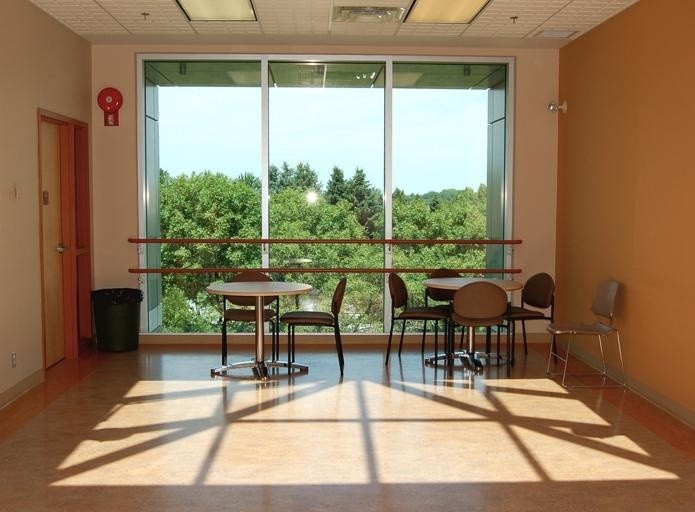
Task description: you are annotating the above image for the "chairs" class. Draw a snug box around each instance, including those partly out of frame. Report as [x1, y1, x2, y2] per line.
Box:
[546, 279, 626, 388]
[385, 268, 558, 376]
[222, 271, 347, 376]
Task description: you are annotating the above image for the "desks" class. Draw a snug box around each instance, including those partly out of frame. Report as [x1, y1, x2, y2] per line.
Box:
[206, 282, 313, 381]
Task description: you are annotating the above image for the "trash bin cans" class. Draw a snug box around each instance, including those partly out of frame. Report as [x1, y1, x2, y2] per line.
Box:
[91, 288, 144, 352]
